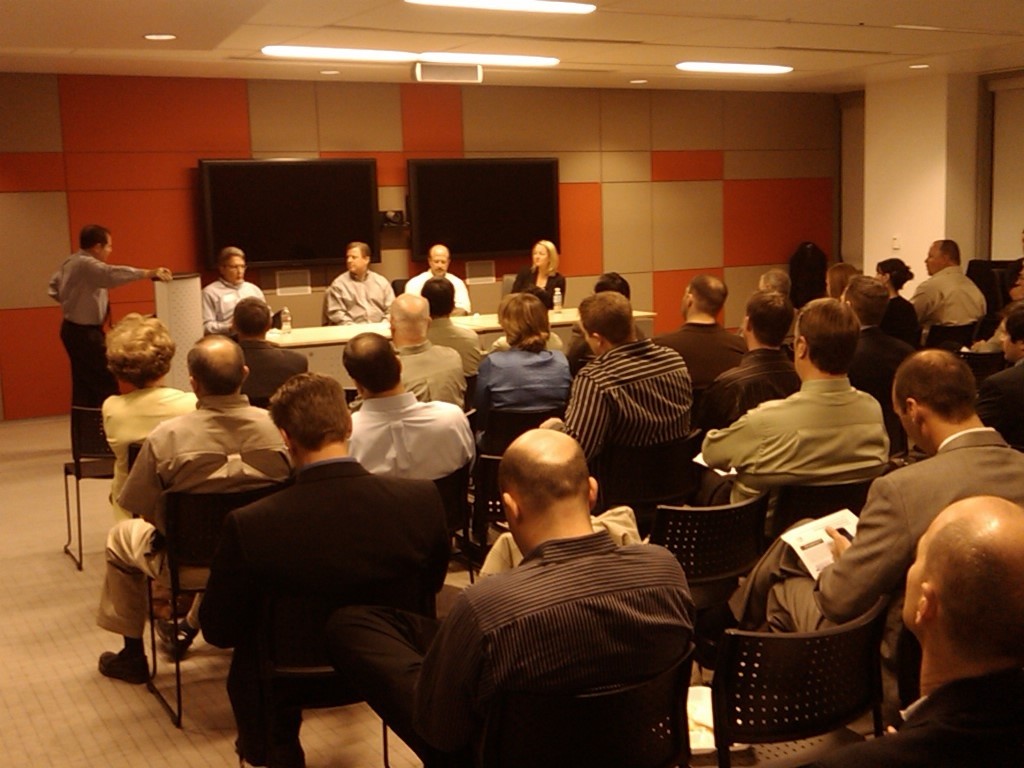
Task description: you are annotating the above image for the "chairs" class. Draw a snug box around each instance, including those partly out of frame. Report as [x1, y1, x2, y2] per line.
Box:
[64, 258, 1023, 768]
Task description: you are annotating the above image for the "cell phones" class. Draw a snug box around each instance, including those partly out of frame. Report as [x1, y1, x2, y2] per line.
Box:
[838, 528, 852, 542]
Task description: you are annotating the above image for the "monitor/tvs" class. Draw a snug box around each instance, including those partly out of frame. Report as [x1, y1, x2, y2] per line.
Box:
[199, 157, 381, 268]
[408, 157, 561, 262]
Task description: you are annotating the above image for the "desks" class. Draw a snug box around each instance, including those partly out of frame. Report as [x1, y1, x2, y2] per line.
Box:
[264, 307, 658, 390]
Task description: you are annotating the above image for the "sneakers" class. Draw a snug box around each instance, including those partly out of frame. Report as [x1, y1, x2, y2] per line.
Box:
[155, 618, 192, 656]
[98, 649, 150, 683]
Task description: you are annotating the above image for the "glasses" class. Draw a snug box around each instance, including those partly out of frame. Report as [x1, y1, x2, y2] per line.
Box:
[787, 339, 796, 350]
[225, 263, 247, 271]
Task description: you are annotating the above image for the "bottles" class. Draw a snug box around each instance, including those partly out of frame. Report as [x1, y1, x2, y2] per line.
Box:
[553, 287, 563, 314]
[281, 306, 292, 334]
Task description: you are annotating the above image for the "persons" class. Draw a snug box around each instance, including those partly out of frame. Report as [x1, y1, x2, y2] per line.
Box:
[811, 496, 1024, 768]
[348, 259, 1024, 540]
[199, 371, 450, 768]
[232, 297, 308, 408]
[511, 240, 566, 310]
[907, 240, 987, 353]
[693, 350, 1024, 729]
[404, 245, 472, 317]
[101, 311, 201, 524]
[202, 247, 274, 345]
[342, 332, 476, 525]
[48, 223, 174, 409]
[324, 242, 397, 328]
[336, 428, 694, 768]
[96, 334, 295, 683]
